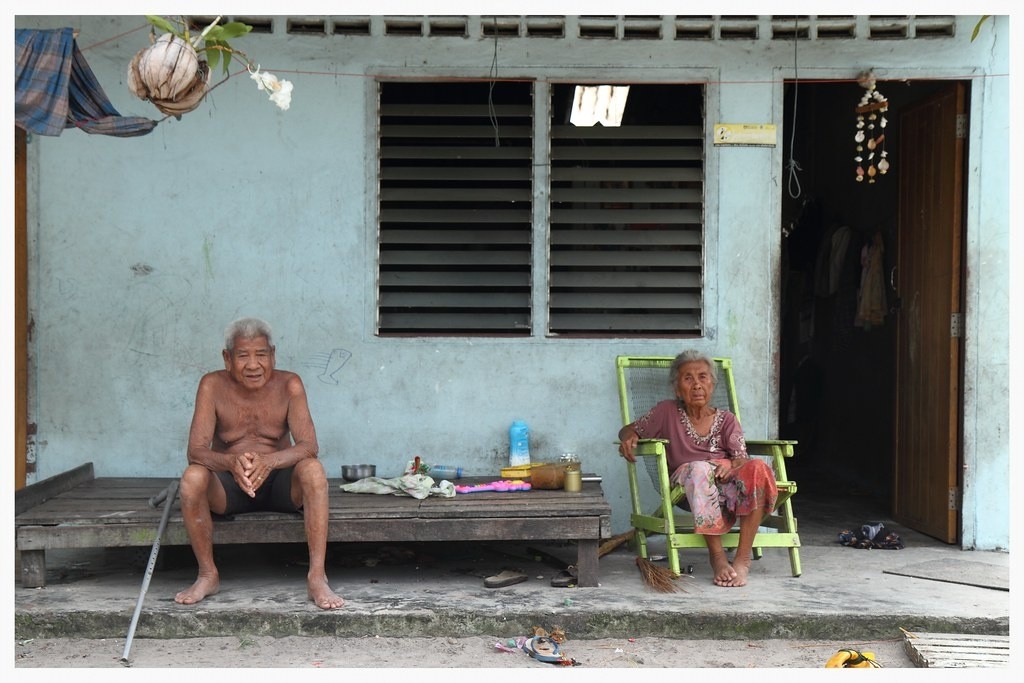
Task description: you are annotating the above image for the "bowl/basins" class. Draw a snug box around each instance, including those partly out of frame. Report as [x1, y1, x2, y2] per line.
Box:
[342, 464, 376, 482]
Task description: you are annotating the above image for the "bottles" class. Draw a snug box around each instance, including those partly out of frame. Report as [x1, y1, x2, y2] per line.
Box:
[508, 420, 530, 467]
[429, 465, 468, 479]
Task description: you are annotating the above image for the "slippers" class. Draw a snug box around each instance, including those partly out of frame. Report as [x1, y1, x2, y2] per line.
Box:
[523, 635, 563, 662]
[551, 568, 578, 587]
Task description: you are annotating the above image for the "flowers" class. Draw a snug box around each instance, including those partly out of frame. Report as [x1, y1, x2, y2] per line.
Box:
[144, 15, 293, 111]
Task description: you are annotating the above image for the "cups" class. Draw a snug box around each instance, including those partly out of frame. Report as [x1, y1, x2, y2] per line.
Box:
[563, 470, 582, 492]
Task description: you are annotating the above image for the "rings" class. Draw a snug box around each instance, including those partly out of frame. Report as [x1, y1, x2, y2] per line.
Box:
[258, 476, 261, 480]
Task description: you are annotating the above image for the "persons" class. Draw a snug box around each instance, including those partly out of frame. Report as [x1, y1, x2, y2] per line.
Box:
[618, 350, 778, 587]
[175, 318, 343, 609]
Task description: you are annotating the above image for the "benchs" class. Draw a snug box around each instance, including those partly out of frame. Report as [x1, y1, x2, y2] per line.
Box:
[15, 463, 614, 587]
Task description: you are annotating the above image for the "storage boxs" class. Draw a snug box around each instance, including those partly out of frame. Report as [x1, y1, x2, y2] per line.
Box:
[501, 463, 542, 477]
[529, 464, 564, 490]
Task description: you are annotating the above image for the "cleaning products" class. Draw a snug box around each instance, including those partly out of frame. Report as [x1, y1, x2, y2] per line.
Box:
[509, 420, 530, 466]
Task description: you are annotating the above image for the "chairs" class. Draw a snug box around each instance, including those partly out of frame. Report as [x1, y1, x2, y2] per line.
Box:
[613, 355, 804, 578]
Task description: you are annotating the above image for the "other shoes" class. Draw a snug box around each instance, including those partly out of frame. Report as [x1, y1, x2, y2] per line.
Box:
[485, 568, 529, 587]
